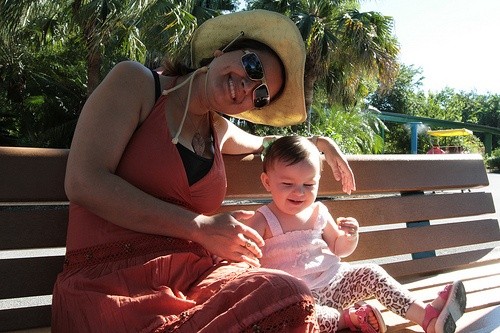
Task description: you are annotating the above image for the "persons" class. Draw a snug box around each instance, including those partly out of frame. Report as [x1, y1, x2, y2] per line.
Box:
[426, 143, 471, 194]
[52, 10, 356, 333]
[243, 136, 466, 333]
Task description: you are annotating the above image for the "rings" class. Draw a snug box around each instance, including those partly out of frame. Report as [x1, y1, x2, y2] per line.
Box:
[245, 240, 252, 247]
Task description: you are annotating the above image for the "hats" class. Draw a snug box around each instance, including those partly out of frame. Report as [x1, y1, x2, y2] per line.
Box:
[191, 9, 307, 127]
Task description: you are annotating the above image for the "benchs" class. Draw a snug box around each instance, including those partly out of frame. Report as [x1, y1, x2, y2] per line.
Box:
[0, 145, 500, 332]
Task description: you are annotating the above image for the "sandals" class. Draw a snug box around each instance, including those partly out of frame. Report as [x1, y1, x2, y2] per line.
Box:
[342, 301, 387, 333]
[422, 279, 466, 333]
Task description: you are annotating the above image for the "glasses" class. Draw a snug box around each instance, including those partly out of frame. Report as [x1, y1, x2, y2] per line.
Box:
[240, 48, 270, 108]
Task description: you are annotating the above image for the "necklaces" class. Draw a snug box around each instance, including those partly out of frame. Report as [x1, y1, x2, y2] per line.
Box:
[174, 91, 206, 157]
